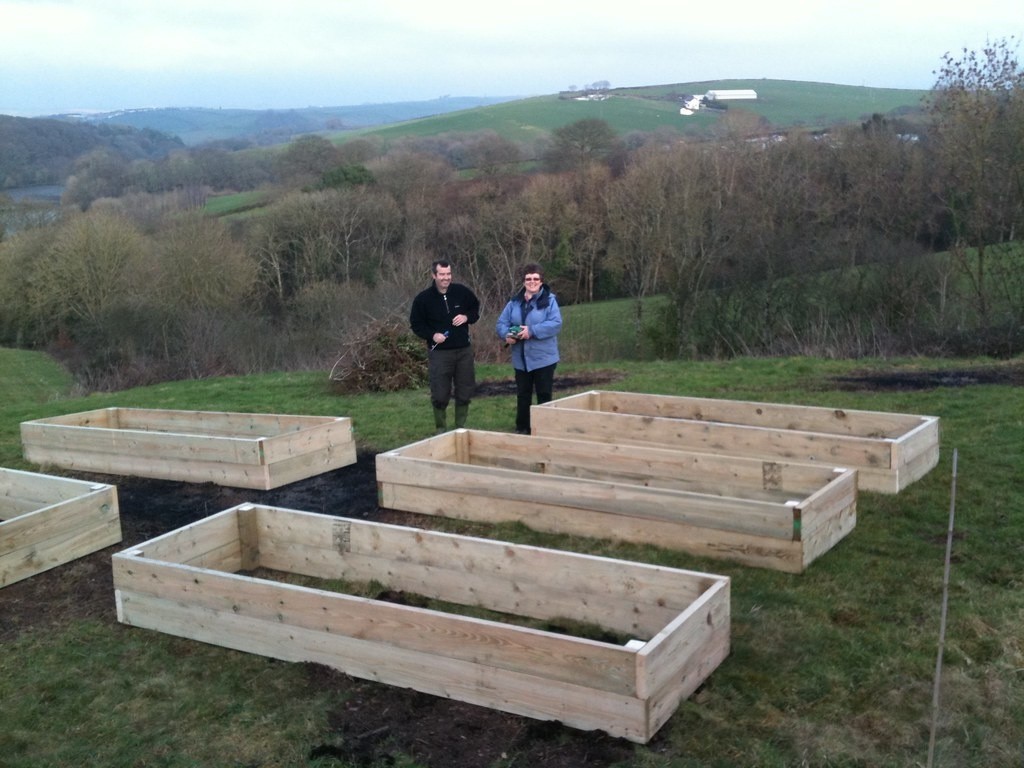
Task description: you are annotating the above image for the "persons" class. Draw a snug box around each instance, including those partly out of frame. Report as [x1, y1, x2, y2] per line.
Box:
[495, 264, 563, 435]
[409, 260, 481, 434]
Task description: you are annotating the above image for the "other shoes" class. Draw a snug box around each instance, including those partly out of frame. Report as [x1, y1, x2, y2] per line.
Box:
[516, 429, 529, 434]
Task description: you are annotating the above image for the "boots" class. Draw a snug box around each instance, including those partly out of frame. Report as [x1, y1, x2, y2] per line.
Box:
[432, 406, 447, 436]
[455, 404, 469, 428]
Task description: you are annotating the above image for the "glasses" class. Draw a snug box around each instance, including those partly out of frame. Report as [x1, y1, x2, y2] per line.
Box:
[525, 278, 539, 281]
